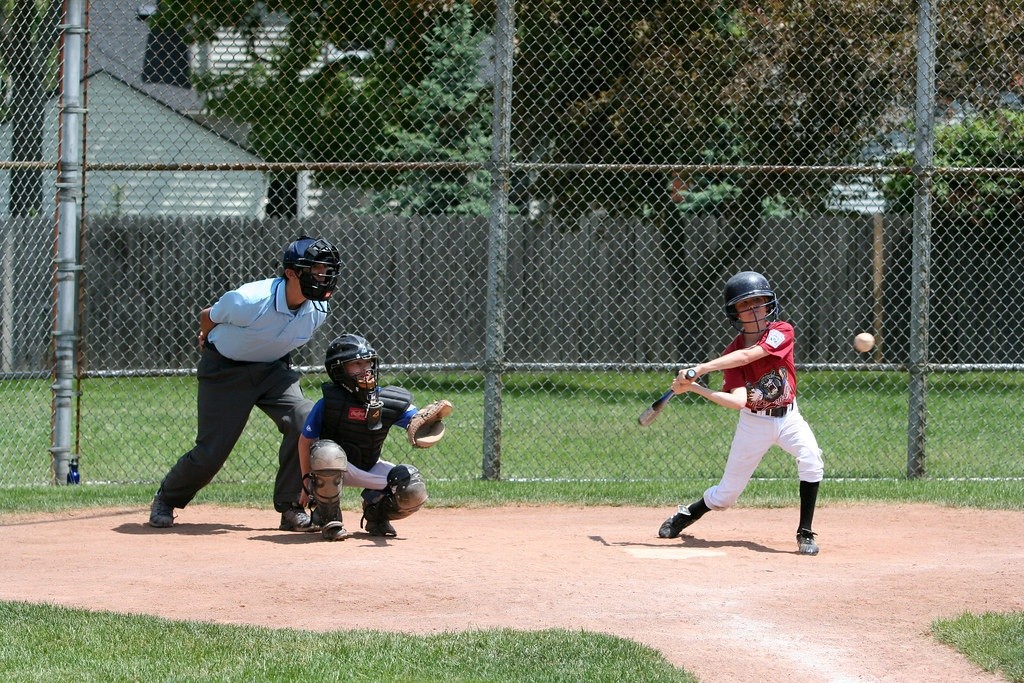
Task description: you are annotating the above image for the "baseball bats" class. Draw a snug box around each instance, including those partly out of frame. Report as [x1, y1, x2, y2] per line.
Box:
[637, 368, 697, 427]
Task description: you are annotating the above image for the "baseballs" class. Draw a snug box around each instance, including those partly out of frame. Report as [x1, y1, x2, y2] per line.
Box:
[853, 332, 876, 353]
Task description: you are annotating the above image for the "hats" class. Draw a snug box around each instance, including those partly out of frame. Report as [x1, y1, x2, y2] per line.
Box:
[282, 236, 340, 266]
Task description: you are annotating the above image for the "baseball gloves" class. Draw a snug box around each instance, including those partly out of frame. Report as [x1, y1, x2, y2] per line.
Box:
[406, 399, 454, 451]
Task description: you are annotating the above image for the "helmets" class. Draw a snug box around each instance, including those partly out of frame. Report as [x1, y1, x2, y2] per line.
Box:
[723, 271, 778, 322]
[324, 334, 380, 404]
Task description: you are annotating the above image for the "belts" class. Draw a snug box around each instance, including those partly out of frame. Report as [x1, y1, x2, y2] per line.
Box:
[751, 404, 794, 418]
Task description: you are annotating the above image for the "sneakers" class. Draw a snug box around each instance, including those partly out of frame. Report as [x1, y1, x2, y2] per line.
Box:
[279, 508, 320, 531]
[360, 501, 397, 536]
[659, 503, 696, 538]
[149, 494, 177, 527]
[321, 522, 347, 540]
[796, 525, 819, 554]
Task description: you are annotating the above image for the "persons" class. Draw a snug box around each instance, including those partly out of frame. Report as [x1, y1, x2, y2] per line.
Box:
[297, 334, 452, 541]
[658, 271, 824, 556]
[149, 235, 342, 532]
